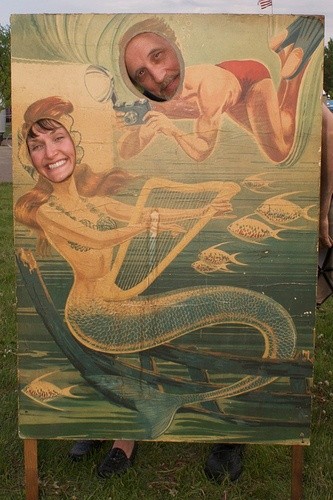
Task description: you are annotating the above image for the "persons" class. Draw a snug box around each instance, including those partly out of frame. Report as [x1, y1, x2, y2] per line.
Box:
[24, 118, 139, 479]
[67, 32, 332, 487]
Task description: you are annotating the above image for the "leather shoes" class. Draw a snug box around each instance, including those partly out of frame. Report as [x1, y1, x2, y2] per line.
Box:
[206, 444, 246, 483]
[68, 441, 99, 462]
[99, 440, 138, 478]
